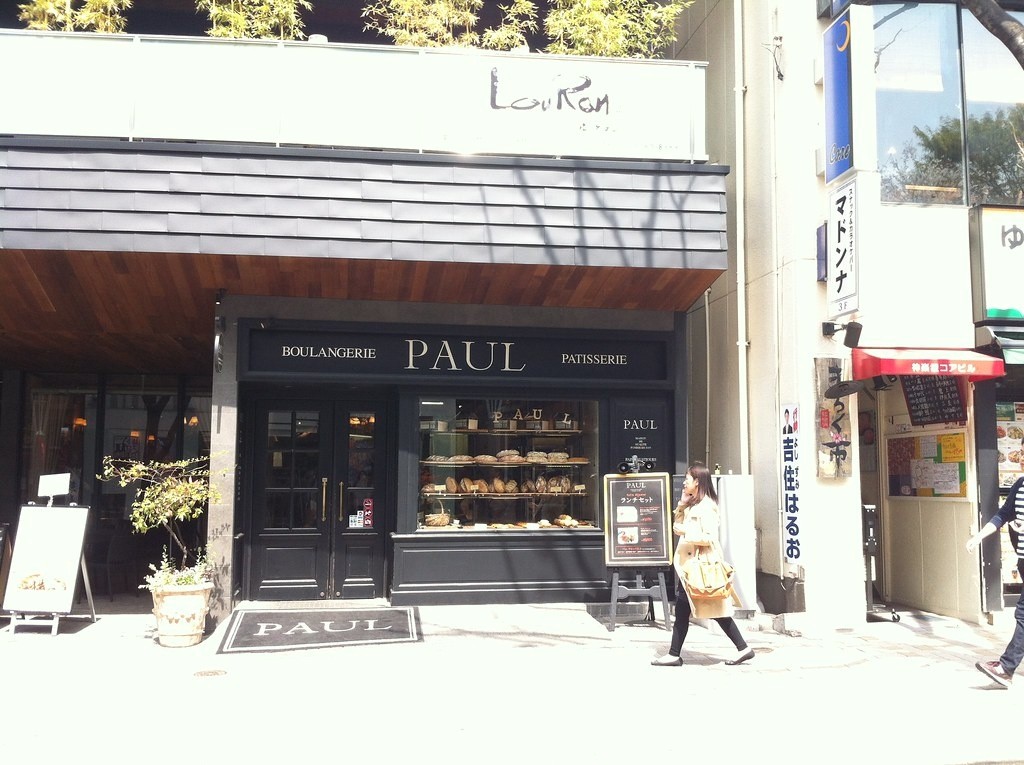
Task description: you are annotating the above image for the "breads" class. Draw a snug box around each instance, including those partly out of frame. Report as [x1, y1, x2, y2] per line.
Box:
[553, 514, 579, 527]
[420, 449, 588, 494]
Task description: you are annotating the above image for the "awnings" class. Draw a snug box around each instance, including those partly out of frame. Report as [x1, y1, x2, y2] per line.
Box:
[852, 348, 1006, 382]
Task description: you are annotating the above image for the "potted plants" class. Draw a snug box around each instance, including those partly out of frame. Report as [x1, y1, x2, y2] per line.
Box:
[94, 446, 238, 647]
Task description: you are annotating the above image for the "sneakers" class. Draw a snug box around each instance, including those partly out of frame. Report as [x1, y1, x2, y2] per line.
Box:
[976, 660, 1013, 688]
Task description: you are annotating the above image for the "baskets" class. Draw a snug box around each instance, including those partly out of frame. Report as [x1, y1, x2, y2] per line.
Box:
[425, 499, 450, 526]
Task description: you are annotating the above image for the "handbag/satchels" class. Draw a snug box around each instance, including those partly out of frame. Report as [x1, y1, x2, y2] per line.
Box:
[683, 537, 734, 600]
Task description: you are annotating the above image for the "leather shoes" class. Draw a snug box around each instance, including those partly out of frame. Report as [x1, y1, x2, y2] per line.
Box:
[650, 657, 684, 666]
[725, 649, 755, 665]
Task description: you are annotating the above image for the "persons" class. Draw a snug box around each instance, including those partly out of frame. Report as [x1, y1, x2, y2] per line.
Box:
[651, 460, 756, 666]
[966, 437, 1024, 688]
[783, 409, 792, 434]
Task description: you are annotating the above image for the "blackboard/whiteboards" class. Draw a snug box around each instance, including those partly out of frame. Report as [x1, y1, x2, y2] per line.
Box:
[899, 375, 968, 427]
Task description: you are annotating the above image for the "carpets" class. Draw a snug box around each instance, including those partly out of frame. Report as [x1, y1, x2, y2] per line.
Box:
[213, 606, 425, 655]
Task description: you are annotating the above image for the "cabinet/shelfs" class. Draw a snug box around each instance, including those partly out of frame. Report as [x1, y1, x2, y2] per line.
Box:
[416, 397, 599, 534]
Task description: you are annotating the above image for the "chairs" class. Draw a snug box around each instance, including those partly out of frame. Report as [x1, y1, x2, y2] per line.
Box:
[89, 533, 140, 601]
